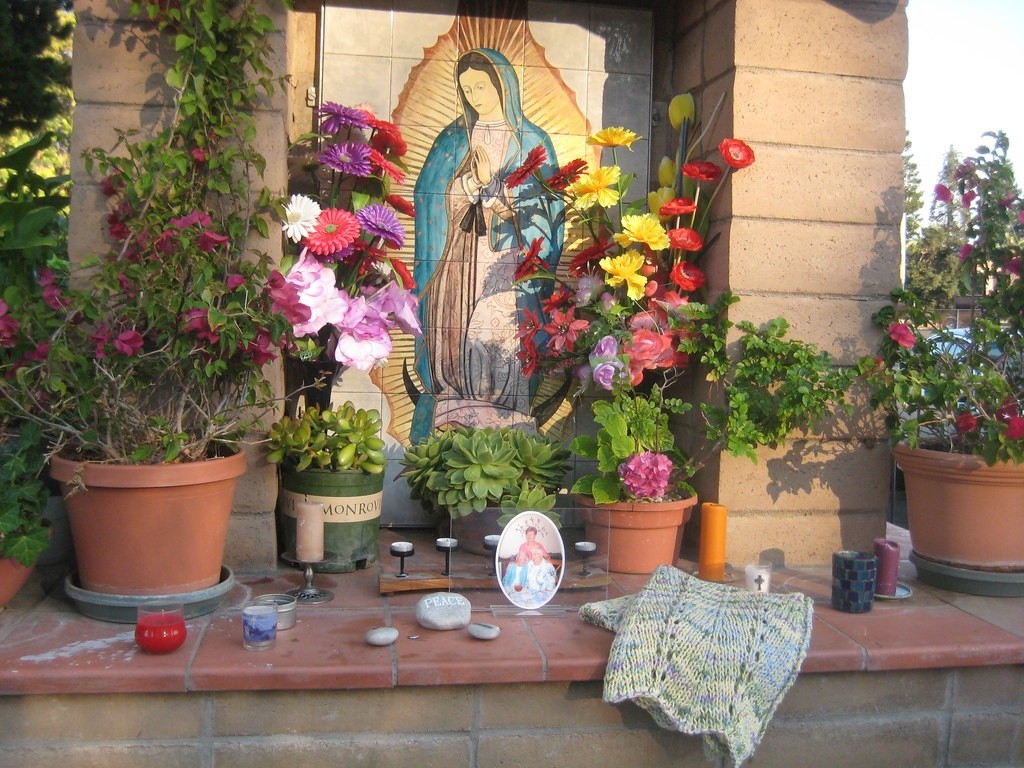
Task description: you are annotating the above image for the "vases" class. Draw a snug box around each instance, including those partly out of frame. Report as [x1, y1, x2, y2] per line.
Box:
[49, 443, 248, 596]
[574, 493, 698, 575]
[892, 435, 1024, 570]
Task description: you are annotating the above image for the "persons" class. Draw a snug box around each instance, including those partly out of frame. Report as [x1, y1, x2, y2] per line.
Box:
[503, 526, 557, 608]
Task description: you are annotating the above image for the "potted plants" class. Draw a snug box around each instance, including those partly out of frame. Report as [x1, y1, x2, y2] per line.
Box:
[265, 400, 385, 574]
[398, 425, 574, 556]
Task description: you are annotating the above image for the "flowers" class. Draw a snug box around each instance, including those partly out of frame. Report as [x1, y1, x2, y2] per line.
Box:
[568, 300, 698, 506]
[870, 130, 1024, 468]
[0, 0, 312, 465]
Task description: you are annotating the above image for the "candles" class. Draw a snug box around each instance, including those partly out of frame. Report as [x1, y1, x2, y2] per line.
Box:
[135, 611, 187, 654]
[699, 503, 727, 583]
[872, 538, 900, 596]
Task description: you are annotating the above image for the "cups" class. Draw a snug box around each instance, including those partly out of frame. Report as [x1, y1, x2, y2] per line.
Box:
[254, 593, 297, 630]
[242, 601, 278, 651]
[135, 601, 186, 654]
[832, 551, 877, 614]
[746, 560, 773, 593]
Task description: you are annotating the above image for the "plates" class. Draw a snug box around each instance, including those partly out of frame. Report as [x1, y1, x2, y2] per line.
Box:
[870, 582, 912, 599]
[495, 511, 564, 608]
